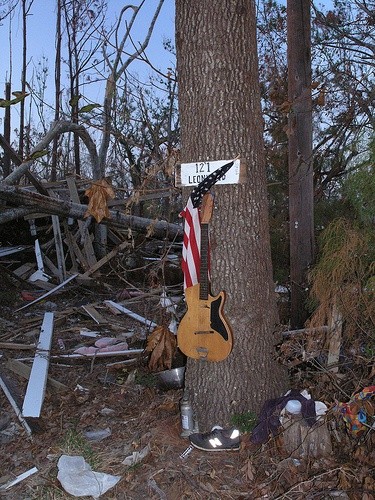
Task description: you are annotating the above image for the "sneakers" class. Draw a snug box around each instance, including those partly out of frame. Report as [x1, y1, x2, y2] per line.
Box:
[190, 428, 242, 451]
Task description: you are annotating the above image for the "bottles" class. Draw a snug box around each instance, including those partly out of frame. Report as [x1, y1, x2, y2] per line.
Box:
[180, 394, 194, 431]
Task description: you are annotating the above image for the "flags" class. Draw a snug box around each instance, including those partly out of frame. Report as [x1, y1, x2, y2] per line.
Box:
[179, 160, 234, 293]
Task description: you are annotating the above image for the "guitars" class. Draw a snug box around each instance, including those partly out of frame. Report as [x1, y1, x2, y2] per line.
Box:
[175, 191, 233, 363]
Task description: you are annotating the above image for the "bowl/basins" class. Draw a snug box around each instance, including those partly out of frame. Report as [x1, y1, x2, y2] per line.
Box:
[154, 366, 186, 391]
